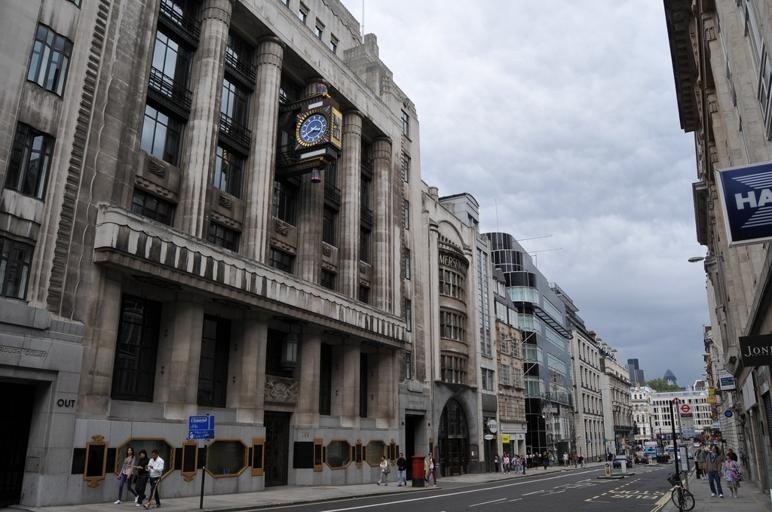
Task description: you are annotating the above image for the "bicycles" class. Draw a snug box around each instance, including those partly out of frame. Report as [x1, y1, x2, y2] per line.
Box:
[667, 471, 696, 512]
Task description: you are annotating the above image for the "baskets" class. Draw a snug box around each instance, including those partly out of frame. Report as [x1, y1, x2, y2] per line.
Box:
[667, 475, 680, 486]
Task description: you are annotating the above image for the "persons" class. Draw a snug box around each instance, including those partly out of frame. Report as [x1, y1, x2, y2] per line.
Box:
[114, 447, 139, 504]
[397, 453, 407, 486]
[143, 448, 165, 509]
[493, 442, 584, 475]
[690, 438, 744, 499]
[424, 454, 431, 486]
[135, 448, 149, 506]
[428, 452, 438, 485]
[376, 455, 388, 487]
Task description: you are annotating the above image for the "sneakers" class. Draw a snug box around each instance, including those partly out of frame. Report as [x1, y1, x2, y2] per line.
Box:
[719, 493, 723, 497]
[114, 500, 121, 504]
[135, 495, 139, 503]
[136, 504, 141, 507]
[711, 493, 717, 497]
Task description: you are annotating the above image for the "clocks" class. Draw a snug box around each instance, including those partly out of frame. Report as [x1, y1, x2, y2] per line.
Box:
[275, 92, 345, 185]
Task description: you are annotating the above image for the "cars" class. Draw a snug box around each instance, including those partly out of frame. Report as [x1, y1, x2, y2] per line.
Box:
[633, 439, 702, 466]
[612, 454, 633, 469]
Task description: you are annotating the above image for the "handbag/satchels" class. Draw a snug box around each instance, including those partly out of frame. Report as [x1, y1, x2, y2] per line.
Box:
[384, 467, 390, 474]
[735, 469, 743, 482]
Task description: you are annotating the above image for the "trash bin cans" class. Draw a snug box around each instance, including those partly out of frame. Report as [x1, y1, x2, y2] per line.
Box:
[412, 456, 425, 487]
